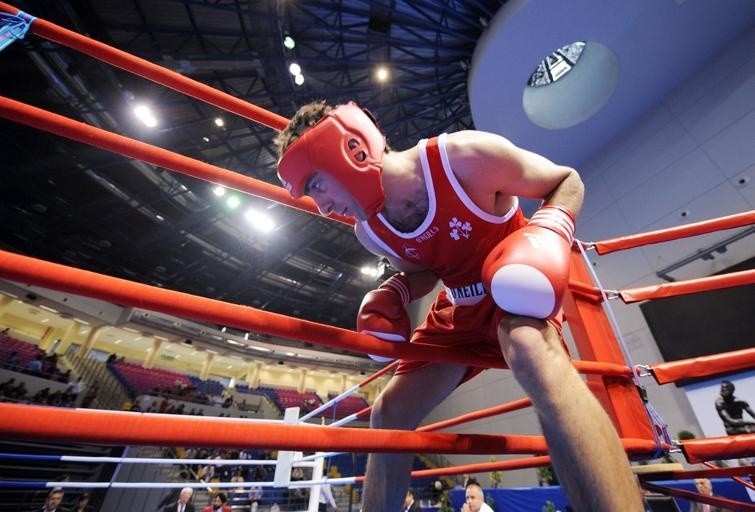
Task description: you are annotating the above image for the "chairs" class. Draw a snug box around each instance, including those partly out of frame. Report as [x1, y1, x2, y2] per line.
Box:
[1, 332, 374, 426]
[405, 452, 455, 508]
[180, 446, 370, 510]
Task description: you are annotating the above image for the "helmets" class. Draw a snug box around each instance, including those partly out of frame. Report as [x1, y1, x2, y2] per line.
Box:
[275, 100, 387, 218]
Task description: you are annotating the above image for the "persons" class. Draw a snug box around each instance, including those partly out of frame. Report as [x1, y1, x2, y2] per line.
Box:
[199, 493, 230, 510]
[129, 384, 215, 416]
[164, 488, 195, 510]
[275, 100, 647, 511]
[72, 493, 96, 510]
[183, 447, 266, 504]
[463, 473, 481, 489]
[42, 487, 69, 509]
[715, 379, 754, 467]
[292, 467, 304, 481]
[460, 484, 496, 511]
[220, 394, 248, 418]
[1, 328, 99, 408]
[318, 469, 337, 510]
[106, 353, 125, 368]
[401, 487, 422, 511]
[430, 479, 444, 505]
[689, 476, 734, 512]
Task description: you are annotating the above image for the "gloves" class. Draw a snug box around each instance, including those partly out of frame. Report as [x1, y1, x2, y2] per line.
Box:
[357, 273, 413, 365]
[480, 203, 575, 322]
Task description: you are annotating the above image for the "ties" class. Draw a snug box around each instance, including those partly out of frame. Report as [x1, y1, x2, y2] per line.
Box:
[180, 503, 184, 512]
[701, 504, 709, 512]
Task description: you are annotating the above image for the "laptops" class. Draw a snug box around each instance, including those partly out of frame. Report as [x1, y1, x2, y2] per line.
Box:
[647, 498, 681, 512]
